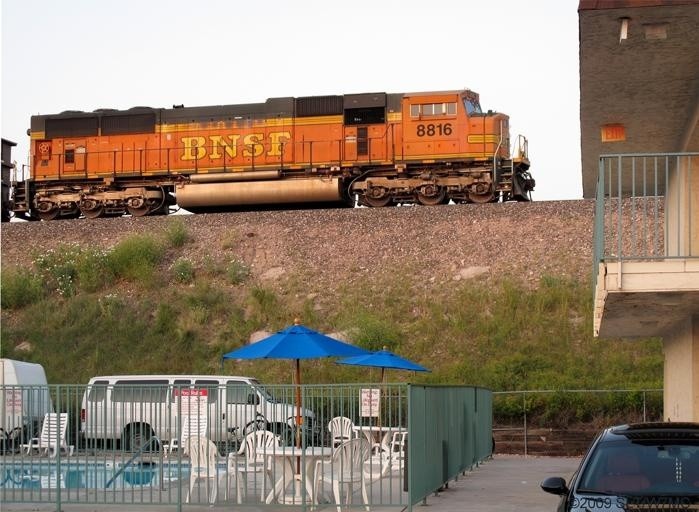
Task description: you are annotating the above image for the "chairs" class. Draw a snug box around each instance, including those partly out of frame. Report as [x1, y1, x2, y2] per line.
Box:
[20, 413, 74, 457]
[163, 416, 409, 507]
[592, 452, 652, 493]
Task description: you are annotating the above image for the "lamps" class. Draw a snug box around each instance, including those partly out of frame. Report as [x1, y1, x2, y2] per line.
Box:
[601, 123, 625, 143]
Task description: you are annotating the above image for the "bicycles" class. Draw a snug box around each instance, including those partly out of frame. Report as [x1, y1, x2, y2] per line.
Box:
[0, 420, 43, 455]
[221, 411, 296, 456]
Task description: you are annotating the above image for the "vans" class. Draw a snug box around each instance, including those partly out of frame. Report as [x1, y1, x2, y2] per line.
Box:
[0, 358, 54, 439]
[80, 375, 315, 451]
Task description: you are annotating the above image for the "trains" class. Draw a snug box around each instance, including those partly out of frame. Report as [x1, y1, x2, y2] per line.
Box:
[0, 89, 535, 221]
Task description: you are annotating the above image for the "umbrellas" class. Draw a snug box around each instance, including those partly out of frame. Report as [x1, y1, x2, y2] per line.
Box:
[334, 344, 433, 426]
[222, 316, 373, 474]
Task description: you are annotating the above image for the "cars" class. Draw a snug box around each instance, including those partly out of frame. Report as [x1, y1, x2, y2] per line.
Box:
[541, 422, 699, 512]
